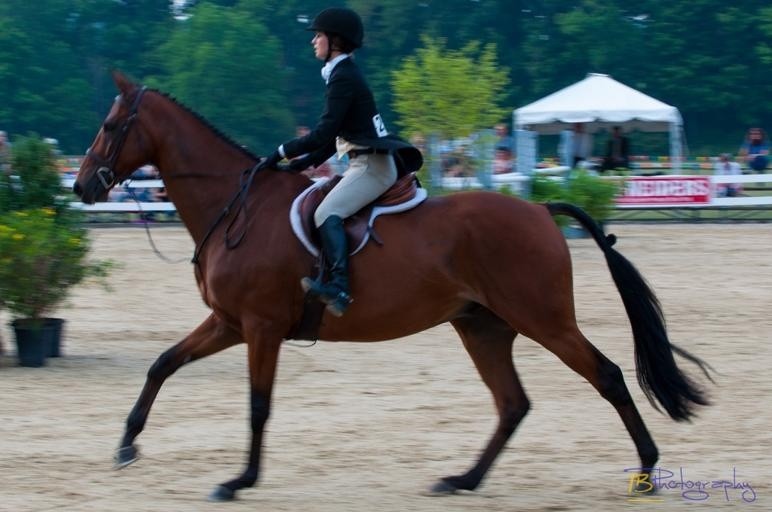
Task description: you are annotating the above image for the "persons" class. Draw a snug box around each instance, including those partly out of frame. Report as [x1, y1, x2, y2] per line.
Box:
[0, 131, 15, 179]
[409, 121, 516, 176]
[712, 153, 743, 197]
[557, 123, 631, 173]
[736, 126, 772, 174]
[265, 8, 423, 318]
[118, 164, 177, 222]
[294, 126, 350, 177]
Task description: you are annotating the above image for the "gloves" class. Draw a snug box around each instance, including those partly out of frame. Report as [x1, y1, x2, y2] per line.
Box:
[266, 151, 284, 168]
[290, 159, 309, 172]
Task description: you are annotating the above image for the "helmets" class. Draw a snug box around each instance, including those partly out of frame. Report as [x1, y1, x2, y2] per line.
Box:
[305, 8, 364, 48]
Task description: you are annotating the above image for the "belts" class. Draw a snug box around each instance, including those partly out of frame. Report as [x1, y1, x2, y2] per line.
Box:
[348, 148, 394, 159]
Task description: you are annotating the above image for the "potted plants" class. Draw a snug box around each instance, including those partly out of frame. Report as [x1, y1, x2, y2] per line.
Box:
[0, 132, 128, 370]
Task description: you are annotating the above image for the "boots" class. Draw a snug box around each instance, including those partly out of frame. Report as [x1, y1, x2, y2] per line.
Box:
[300, 215, 351, 318]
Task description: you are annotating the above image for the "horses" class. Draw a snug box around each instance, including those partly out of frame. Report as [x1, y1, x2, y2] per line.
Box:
[71, 70, 720, 503]
